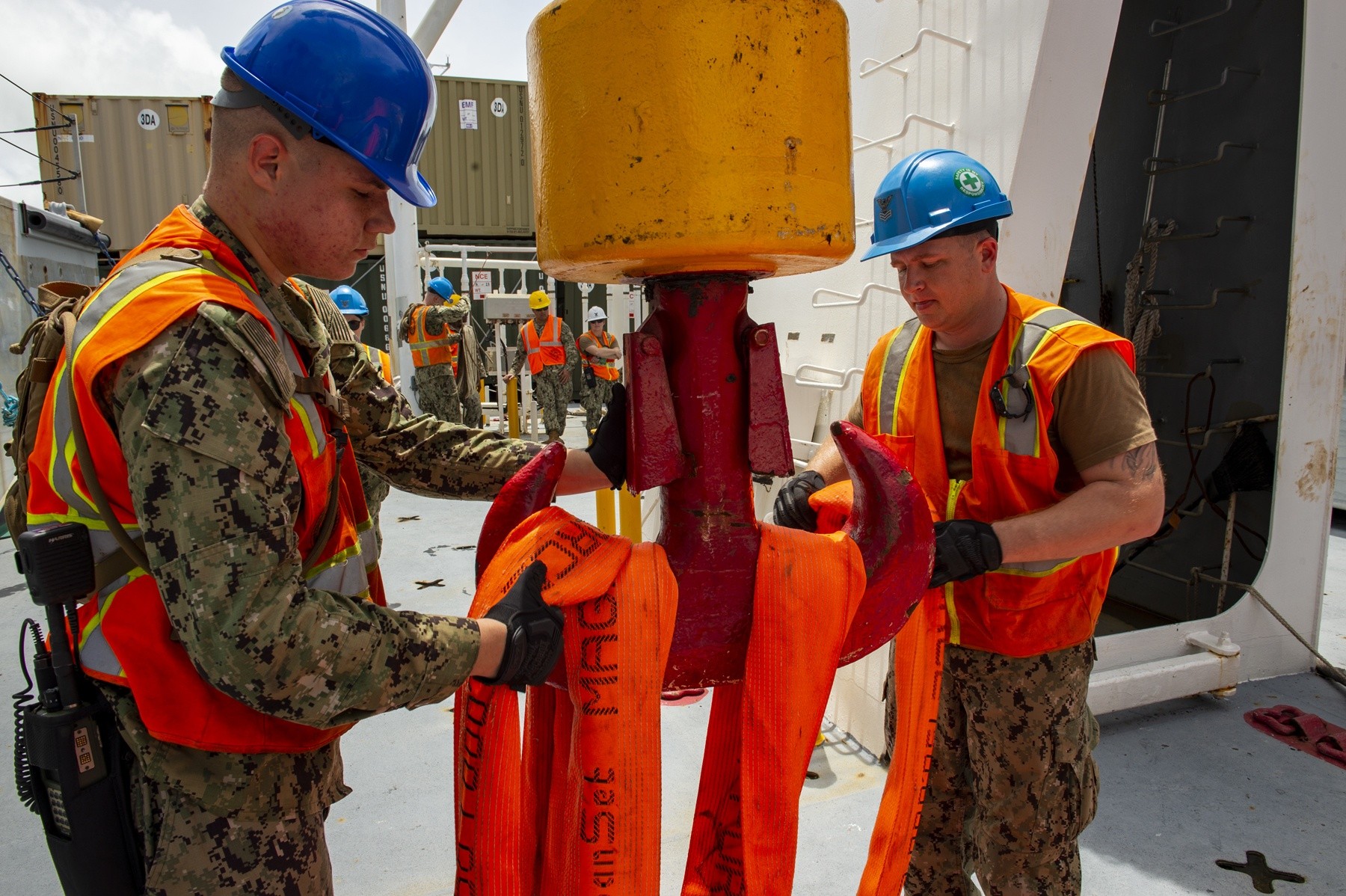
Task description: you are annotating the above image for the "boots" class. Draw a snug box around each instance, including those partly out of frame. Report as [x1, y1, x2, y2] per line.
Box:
[588, 435, 593, 446]
[542, 431, 565, 444]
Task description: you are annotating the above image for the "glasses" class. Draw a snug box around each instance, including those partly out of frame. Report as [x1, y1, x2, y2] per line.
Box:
[591, 320, 605, 324]
[534, 306, 548, 312]
[348, 320, 362, 331]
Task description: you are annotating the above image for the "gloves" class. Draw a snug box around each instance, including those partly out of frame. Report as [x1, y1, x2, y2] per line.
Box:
[585, 382, 628, 490]
[471, 561, 563, 692]
[773, 471, 825, 533]
[928, 520, 1003, 589]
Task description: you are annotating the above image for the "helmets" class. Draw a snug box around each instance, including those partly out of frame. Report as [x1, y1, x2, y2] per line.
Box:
[428, 277, 453, 303]
[859, 149, 1013, 263]
[329, 285, 369, 316]
[220, 0, 437, 207]
[529, 291, 550, 309]
[587, 306, 609, 323]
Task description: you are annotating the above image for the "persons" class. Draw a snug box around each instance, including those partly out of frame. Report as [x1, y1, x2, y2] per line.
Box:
[503, 290, 580, 445]
[575, 306, 622, 446]
[407, 277, 487, 428]
[773, 151, 1165, 896]
[320, 278, 396, 605]
[17, 0, 635, 896]
